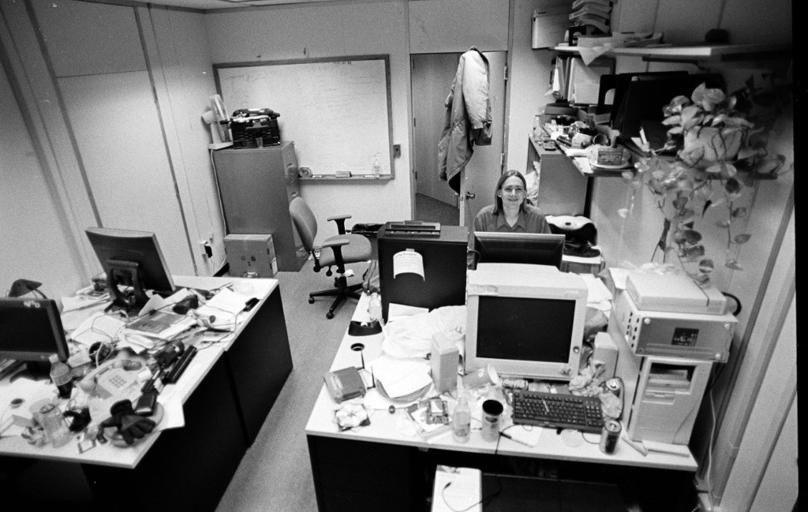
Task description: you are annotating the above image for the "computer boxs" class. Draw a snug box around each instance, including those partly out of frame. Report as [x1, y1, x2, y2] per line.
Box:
[606, 309, 713, 445]
[377, 223, 468, 326]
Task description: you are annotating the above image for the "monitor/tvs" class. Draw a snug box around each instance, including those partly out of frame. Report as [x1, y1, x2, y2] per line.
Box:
[474, 231, 565, 269]
[85, 227, 177, 316]
[464, 263, 588, 381]
[0, 298, 69, 384]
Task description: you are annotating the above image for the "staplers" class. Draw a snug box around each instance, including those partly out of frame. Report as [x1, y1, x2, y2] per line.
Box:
[242, 297, 259, 312]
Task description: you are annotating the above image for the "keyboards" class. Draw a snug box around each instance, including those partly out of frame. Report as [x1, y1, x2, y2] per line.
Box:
[513, 389, 604, 432]
[152, 286, 210, 305]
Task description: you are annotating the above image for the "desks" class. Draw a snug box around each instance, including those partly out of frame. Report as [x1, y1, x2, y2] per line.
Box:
[0, 346, 247, 511]
[305, 262, 699, 512]
[60, 273, 294, 446]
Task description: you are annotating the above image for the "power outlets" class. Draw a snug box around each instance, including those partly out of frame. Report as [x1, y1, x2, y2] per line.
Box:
[201, 241, 210, 256]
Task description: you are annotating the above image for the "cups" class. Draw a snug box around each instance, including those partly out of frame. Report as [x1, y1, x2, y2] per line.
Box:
[481, 398, 504, 442]
[27, 400, 75, 448]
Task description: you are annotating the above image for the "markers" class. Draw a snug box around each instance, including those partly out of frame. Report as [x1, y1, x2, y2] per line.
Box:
[362, 175, 379, 178]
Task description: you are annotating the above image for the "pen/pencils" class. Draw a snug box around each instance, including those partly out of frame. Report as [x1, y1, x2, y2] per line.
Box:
[496, 431, 536, 449]
[311, 175, 323, 178]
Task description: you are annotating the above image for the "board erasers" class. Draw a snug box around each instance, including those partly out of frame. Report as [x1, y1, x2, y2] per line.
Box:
[335, 171, 350, 178]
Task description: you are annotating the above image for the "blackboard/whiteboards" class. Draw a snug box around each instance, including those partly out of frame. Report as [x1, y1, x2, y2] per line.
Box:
[211, 52, 395, 186]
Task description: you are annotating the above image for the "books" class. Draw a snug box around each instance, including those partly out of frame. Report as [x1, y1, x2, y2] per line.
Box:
[568, 0, 613, 34]
[562, 56, 616, 105]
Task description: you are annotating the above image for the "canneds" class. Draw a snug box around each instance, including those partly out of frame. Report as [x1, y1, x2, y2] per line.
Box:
[605, 378, 620, 397]
[38, 402, 72, 449]
[599, 420, 622, 454]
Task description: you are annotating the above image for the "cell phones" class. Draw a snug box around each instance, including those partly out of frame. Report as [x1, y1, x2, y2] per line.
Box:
[135, 393, 156, 417]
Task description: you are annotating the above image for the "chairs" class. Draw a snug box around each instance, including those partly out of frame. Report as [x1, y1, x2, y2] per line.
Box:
[288, 196, 372, 319]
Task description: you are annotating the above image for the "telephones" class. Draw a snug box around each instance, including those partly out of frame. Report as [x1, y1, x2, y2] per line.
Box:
[79, 358, 144, 403]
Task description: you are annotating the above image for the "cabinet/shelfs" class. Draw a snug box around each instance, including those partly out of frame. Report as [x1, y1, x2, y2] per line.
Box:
[212, 139, 311, 272]
[525, 133, 588, 217]
[538, 42, 777, 177]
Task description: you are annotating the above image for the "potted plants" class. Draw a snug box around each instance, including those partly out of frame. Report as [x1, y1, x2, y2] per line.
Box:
[616, 83, 787, 288]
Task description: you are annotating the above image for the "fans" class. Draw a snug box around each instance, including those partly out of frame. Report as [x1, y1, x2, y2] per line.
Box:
[201, 94, 234, 151]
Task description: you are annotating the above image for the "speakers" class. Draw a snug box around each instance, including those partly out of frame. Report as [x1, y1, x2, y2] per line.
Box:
[593, 332, 618, 381]
[431, 334, 459, 392]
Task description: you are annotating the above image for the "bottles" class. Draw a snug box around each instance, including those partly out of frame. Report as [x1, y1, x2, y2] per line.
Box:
[451, 396, 469, 445]
[366, 291, 382, 323]
[47, 352, 77, 401]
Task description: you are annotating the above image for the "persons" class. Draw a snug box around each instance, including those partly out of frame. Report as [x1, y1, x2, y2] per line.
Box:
[466, 169, 552, 270]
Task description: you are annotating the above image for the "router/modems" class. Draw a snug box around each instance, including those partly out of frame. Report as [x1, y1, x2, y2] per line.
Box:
[322, 366, 366, 403]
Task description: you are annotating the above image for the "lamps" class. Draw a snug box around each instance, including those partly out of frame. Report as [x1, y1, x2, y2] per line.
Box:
[9, 278, 68, 337]
